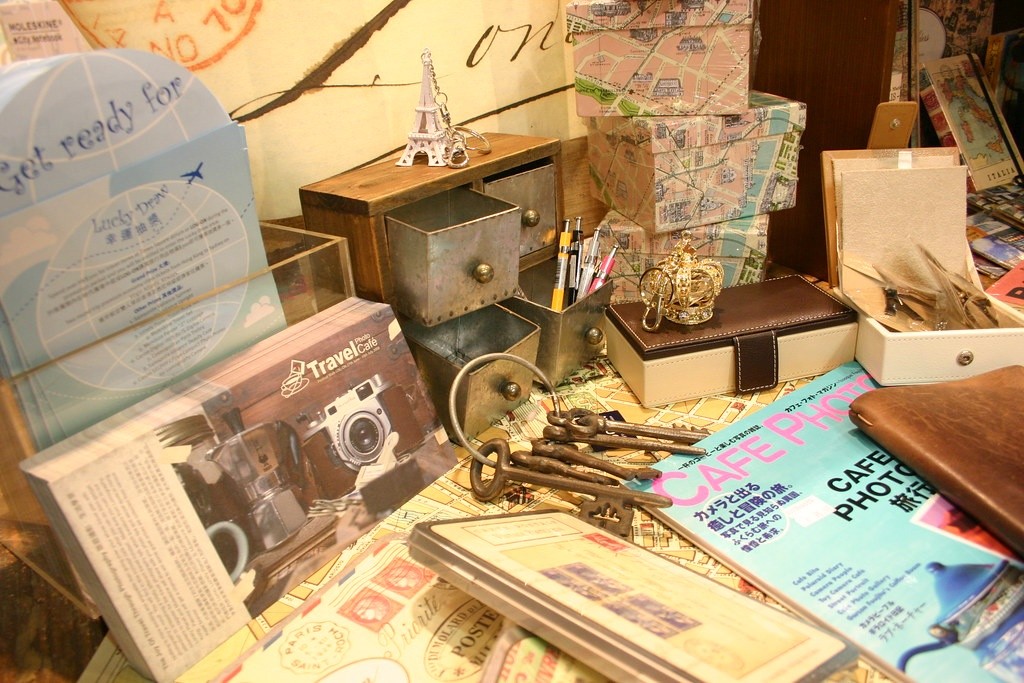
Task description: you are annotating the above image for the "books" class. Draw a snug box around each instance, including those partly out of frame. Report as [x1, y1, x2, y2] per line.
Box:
[615, 359, 1024, 683]
[917, 27, 1023, 328]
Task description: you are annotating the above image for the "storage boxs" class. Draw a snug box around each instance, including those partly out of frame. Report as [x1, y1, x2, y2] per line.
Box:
[18, 295, 460, 683]
[565, 0, 761, 118]
[602, 273, 860, 409]
[406, 508, 862, 683]
[0, 220, 358, 619]
[580, 90, 807, 235]
[820, 100, 1024, 387]
[596, 207, 770, 304]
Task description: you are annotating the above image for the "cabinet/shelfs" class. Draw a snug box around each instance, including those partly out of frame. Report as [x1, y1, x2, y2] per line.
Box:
[299, 131, 615, 447]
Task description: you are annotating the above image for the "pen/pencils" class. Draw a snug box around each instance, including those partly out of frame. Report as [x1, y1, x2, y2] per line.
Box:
[576, 227, 601, 301]
[571, 216, 584, 304]
[587, 245, 620, 296]
[551, 218, 572, 310]
